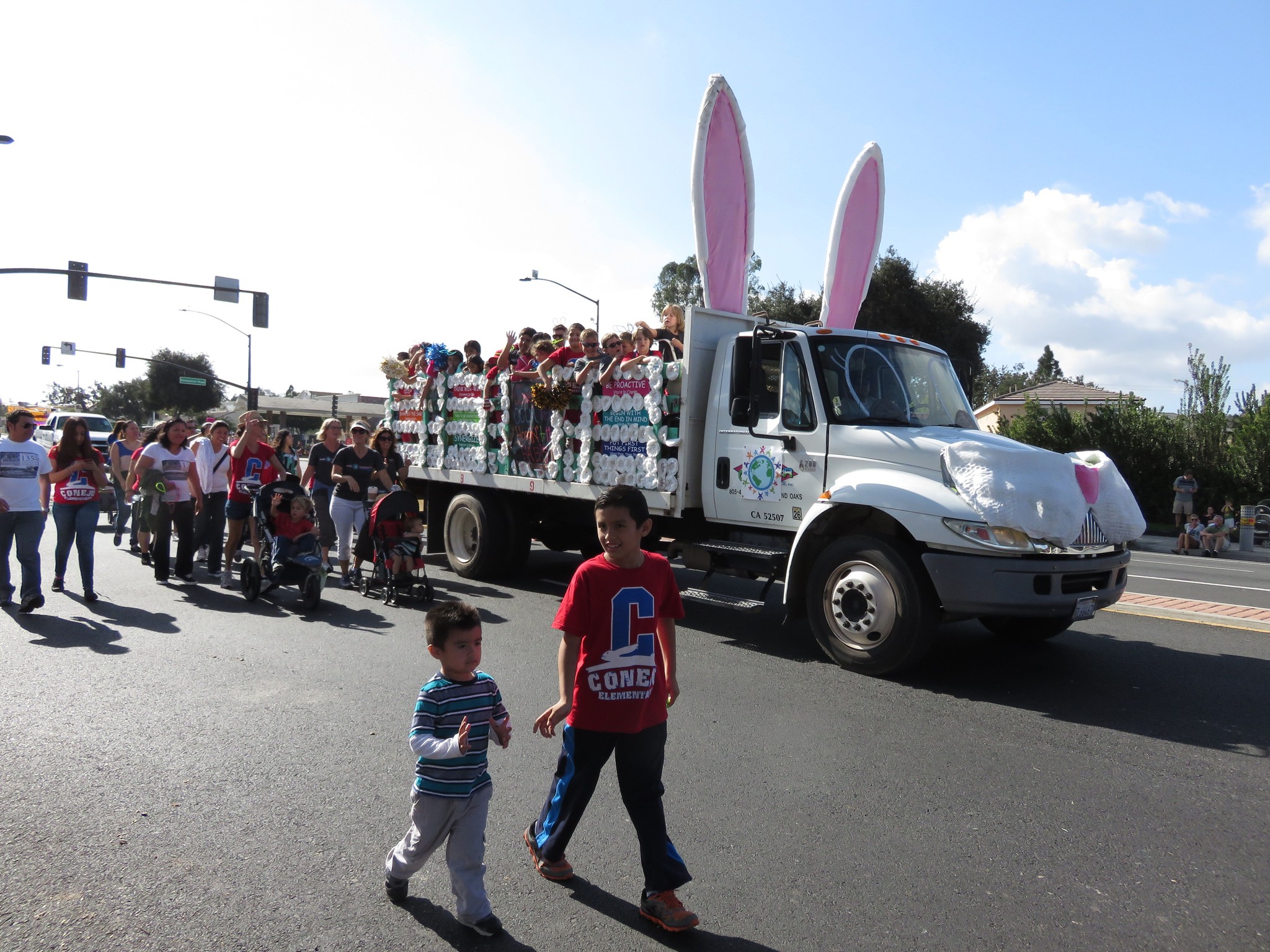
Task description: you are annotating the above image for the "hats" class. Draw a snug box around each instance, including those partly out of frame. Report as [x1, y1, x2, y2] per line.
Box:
[237, 410, 268, 428]
[349, 420, 371, 433]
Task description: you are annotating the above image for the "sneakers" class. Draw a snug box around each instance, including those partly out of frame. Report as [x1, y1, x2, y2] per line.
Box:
[639, 887, 699, 932]
[384, 868, 408, 905]
[114, 526, 273, 595]
[0, 590, 13, 607]
[52, 574, 65, 593]
[1211, 551, 1218, 558]
[18, 593, 45, 613]
[339, 563, 363, 590]
[523, 819, 573, 880]
[457, 911, 502, 936]
[387, 572, 419, 586]
[318, 561, 333, 574]
[1202, 550, 1211, 557]
[272, 561, 282, 576]
[83, 589, 98, 600]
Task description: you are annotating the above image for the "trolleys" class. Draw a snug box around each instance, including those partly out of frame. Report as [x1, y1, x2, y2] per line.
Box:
[98, 472, 118, 522]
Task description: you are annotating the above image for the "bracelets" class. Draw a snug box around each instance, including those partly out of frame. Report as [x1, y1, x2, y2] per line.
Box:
[41, 507, 50, 513]
[67, 465, 74, 476]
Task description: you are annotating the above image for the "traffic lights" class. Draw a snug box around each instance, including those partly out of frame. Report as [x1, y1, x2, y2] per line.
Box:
[116, 348, 126, 368]
[42, 346, 51, 365]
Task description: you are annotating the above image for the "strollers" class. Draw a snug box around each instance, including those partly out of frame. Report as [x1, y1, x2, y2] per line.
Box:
[240, 475, 325, 612]
[359, 484, 435, 606]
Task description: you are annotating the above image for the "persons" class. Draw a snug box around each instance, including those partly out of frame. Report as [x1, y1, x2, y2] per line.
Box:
[1171, 469, 1198, 532]
[524, 484, 699, 932]
[1171, 515, 1205, 555]
[831, 369, 903, 420]
[1220, 499, 1239, 529]
[1203, 507, 1218, 528]
[384, 600, 512, 936]
[109, 410, 424, 594]
[0, 410, 52, 612]
[49, 416, 107, 601]
[399, 304, 684, 409]
[1200, 516, 1230, 558]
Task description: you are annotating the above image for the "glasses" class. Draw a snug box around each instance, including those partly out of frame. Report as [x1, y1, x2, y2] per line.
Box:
[1191, 519, 1198, 521]
[254, 422, 266, 427]
[186, 426, 197, 430]
[379, 435, 395, 441]
[329, 426, 342, 431]
[16, 422, 37, 430]
[351, 429, 366, 435]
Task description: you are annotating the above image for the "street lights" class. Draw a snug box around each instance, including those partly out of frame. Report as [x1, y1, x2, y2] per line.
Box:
[519, 276, 600, 337]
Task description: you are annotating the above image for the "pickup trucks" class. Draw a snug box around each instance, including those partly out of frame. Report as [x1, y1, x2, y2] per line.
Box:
[33, 412, 115, 462]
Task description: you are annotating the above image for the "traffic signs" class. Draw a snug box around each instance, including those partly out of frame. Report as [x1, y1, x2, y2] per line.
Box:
[61, 342, 76, 356]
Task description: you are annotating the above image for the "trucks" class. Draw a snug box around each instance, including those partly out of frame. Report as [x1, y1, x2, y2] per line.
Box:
[394, 306, 1132, 673]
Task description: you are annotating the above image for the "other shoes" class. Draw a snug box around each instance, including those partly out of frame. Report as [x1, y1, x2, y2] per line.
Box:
[1171, 549, 1180, 555]
[1183, 550, 1189, 555]
